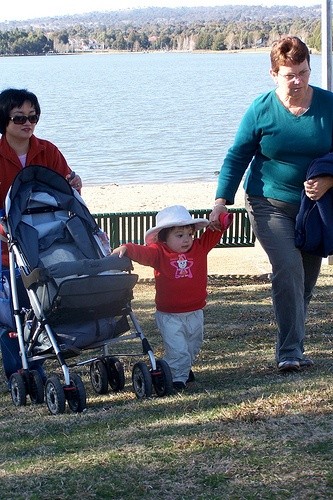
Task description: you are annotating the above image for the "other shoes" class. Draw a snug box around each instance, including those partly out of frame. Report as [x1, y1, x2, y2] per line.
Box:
[173, 370, 196, 389]
[277, 358, 314, 371]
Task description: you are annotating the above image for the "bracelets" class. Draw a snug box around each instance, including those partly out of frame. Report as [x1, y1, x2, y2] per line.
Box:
[214, 203, 227, 210]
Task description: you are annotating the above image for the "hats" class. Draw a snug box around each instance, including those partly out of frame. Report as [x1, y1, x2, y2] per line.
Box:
[145, 205, 209, 244]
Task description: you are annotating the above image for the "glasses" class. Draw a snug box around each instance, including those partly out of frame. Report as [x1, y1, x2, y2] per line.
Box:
[10, 115, 38, 124]
[278, 68, 311, 80]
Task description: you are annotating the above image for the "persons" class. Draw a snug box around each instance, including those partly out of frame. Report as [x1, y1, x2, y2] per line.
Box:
[210, 37, 333, 371]
[0, 87, 83, 392]
[111, 206, 234, 390]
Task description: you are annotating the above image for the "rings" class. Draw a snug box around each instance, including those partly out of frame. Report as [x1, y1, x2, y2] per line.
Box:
[212, 220, 215, 223]
[77, 183, 80, 187]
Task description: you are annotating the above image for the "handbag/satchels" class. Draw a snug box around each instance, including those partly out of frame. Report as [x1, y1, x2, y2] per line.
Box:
[0, 268, 31, 331]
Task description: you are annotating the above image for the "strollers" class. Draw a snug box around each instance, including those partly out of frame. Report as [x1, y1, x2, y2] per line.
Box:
[0, 165, 172, 415]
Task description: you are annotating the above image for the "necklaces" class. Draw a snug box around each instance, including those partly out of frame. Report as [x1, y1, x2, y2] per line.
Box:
[295, 100, 306, 115]
